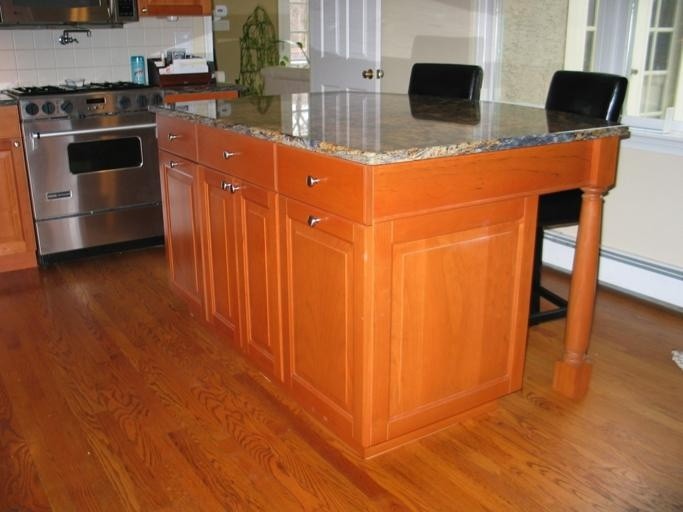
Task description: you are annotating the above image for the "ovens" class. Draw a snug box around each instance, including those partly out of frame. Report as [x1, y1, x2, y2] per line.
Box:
[21, 112, 164, 268]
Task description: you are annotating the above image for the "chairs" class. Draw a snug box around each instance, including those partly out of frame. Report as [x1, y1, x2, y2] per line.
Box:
[407, 61, 485, 101]
[528, 70, 631, 326]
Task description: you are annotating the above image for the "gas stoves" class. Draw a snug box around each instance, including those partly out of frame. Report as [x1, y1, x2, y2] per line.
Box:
[1, 79, 164, 121]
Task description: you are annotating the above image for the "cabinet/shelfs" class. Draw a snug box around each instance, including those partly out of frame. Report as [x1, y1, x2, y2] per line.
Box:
[155, 114, 620, 463]
[0, 106, 39, 274]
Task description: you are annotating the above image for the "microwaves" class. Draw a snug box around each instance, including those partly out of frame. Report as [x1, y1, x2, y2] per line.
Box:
[0, 0, 138, 30]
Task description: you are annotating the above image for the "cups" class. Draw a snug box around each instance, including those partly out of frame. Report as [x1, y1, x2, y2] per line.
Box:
[131, 55, 145, 83]
